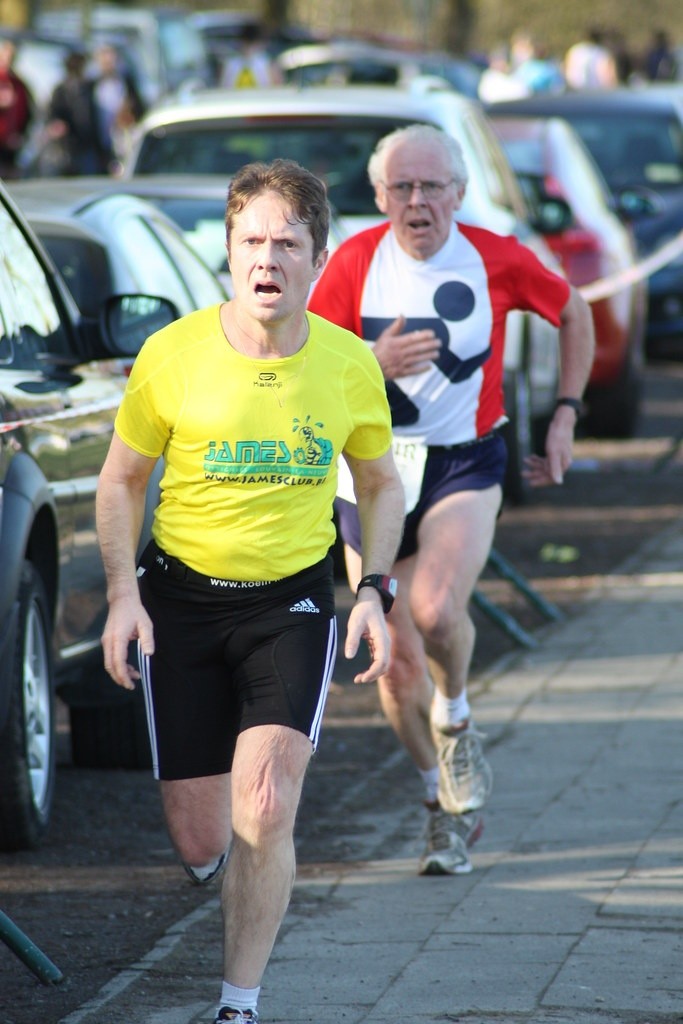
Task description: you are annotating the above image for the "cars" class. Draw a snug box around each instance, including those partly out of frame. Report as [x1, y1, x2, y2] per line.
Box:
[22, 170, 237, 356]
[488, 85, 682, 359]
[479, 114, 655, 435]
[2, 184, 183, 860]
[0, 5, 486, 134]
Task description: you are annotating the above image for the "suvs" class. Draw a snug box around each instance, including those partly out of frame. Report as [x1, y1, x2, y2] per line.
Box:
[122, 74, 574, 509]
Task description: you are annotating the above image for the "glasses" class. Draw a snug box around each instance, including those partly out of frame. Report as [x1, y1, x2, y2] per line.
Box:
[378, 179, 454, 200]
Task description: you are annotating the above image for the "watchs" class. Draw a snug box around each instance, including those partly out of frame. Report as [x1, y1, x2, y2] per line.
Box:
[356, 574, 397, 614]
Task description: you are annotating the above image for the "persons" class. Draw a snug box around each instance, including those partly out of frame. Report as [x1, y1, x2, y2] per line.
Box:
[216, 23, 276, 90]
[0, 36, 143, 181]
[96, 159, 404, 1024]
[306, 124, 595, 876]
[477, 27, 676, 104]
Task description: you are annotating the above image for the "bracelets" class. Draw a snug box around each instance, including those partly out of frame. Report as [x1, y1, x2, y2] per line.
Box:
[554, 397, 584, 420]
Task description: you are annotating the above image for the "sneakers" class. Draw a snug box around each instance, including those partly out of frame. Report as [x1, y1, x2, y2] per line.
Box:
[181, 846, 230, 884]
[213, 1005, 259, 1024]
[427, 697, 493, 816]
[417, 800, 483, 874]
